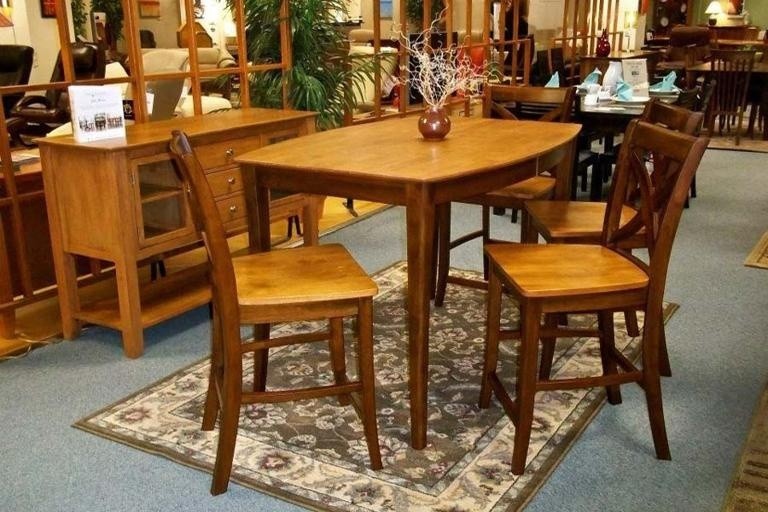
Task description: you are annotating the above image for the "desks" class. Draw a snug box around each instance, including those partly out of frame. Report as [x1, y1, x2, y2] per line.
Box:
[233, 113, 585, 449]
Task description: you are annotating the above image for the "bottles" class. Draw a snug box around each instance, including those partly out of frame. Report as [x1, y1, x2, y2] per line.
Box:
[596, 28, 610, 57]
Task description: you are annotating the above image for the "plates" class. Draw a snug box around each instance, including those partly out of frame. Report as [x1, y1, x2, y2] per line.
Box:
[611, 94, 650, 103]
[648, 87, 678, 95]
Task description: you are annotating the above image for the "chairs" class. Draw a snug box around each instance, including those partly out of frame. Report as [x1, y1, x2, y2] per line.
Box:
[171, 123, 386, 501]
[649, 0, 767, 146]
[343, 27, 479, 110]
[434, 78, 578, 326]
[483, 33, 716, 209]
[464, 120, 713, 473]
[1, 19, 238, 147]
[518, 98, 711, 381]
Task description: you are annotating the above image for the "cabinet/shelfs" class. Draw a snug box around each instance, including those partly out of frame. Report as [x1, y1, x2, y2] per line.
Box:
[33, 105, 322, 359]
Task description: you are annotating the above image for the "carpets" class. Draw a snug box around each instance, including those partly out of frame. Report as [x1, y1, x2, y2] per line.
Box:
[68, 258, 679, 511]
[743, 227, 768, 270]
[718, 376, 767, 511]
